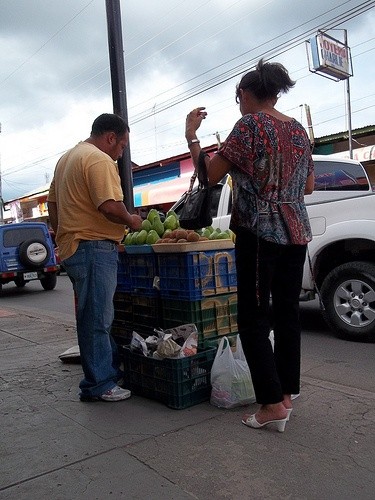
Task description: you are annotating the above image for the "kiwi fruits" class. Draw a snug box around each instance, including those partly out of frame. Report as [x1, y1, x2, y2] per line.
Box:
[156, 229, 209, 244]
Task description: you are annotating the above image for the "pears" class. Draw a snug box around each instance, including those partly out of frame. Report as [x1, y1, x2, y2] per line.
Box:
[124, 209, 181, 244]
[201, 226, 231, 239]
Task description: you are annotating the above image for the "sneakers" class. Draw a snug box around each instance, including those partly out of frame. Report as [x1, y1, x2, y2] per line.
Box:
[101, 385, 132, 401]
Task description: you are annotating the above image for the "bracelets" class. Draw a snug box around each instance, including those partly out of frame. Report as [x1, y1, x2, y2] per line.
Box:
[187, 139, 200, 148]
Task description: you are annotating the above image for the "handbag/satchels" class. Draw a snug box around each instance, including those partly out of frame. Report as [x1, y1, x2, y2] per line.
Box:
[181, 149, 213, 229]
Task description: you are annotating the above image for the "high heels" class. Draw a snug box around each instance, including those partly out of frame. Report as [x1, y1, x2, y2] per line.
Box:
[241, 408, 294, 434]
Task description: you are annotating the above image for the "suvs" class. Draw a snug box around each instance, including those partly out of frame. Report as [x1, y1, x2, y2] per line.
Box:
[0, 222, 60, 291]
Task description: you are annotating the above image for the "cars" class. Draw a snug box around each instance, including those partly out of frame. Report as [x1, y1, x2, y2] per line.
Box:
[156, 155, 375, 342]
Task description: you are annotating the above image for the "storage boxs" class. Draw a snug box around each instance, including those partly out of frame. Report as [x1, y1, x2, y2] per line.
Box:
[117, 344, 217, 410]
[112, 249, 240, 410]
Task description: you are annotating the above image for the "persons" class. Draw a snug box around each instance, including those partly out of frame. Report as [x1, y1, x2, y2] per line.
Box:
[47, 113, 143, 401]
[185, 59, 315, 433]
